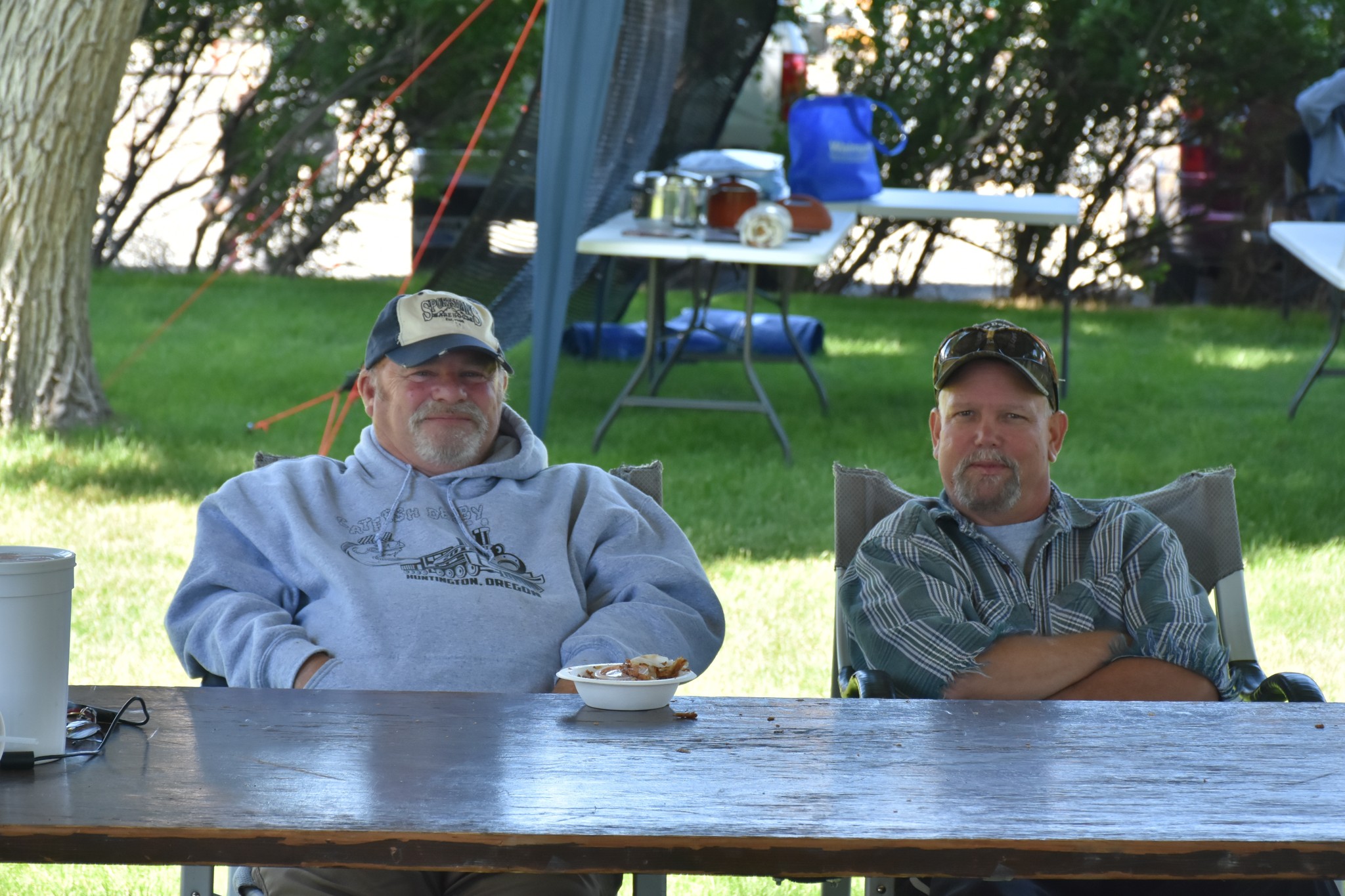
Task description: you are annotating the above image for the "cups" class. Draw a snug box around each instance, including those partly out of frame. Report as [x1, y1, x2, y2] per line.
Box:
[0, 545, 77, 764]
[0, 713, 6, 762]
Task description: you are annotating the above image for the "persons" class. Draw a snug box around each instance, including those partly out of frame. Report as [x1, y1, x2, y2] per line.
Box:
[1297, 69, 1345, 310]
[162, 291, 725, 896]
[835, 319, 1342, 896]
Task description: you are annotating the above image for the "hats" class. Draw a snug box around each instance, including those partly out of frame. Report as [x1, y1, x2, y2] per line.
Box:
[366, 289, 513, 376]
[932, 318, 1057, 416]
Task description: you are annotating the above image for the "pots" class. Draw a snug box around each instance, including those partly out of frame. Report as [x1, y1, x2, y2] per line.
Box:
[624, 160, 835, 231]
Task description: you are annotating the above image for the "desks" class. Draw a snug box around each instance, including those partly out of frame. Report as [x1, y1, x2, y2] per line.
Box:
[574, 212, 856, 465]
[0, 685, 1345, 896]
[1270, 222, 1345, 419]
[827, 188, 1082, 404]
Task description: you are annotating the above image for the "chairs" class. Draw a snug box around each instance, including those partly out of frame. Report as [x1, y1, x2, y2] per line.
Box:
[181, 453, 661, 896]
[831, 459, 1260, 896]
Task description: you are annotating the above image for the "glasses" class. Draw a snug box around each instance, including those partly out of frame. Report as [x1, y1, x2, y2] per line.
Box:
[935, 326, 1059, 411]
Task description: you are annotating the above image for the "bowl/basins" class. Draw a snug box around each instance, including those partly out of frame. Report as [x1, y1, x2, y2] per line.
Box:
[594, 664, 649, 681]
[556, 663, 697, 711]
